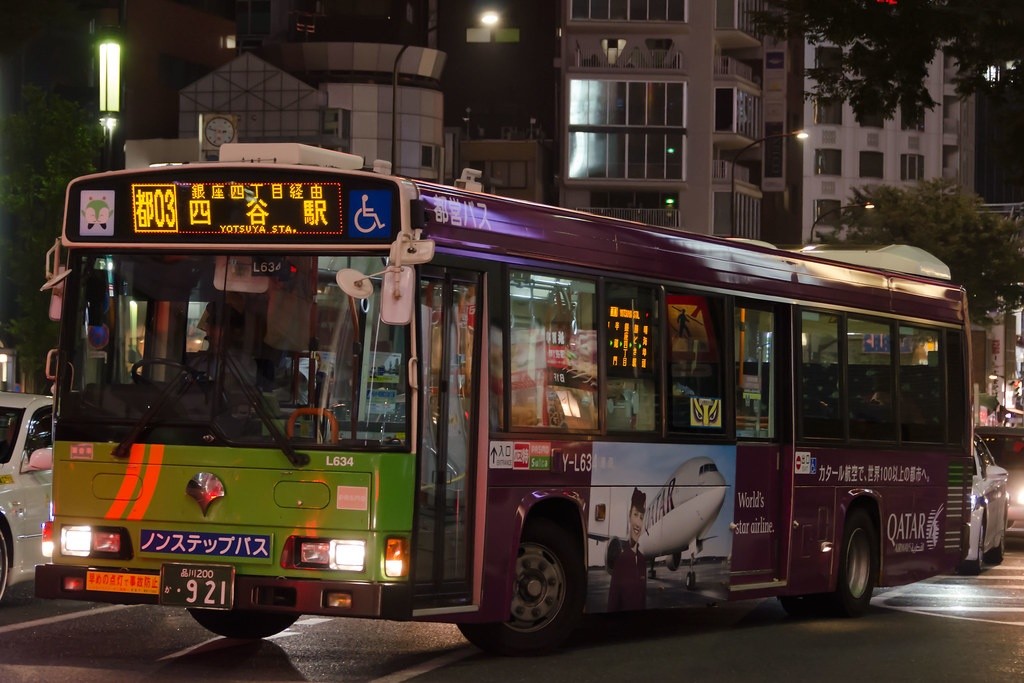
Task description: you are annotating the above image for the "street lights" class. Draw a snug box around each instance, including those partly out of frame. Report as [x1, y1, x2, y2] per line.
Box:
[989, 374, 1006, 408]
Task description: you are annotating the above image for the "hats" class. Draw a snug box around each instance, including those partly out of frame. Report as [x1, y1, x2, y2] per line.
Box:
[631, 486, 646, 513]
[206, 301, 241, 326]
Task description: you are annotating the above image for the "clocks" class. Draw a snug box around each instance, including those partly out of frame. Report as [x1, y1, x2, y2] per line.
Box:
[203, 114, 237, 152]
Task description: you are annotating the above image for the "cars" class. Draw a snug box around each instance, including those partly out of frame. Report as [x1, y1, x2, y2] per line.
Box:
[0, 392, 66, 609]
[956, 425, 1024, 578]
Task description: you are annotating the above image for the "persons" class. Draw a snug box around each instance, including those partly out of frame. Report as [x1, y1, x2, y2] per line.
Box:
[176, 303, 258, 418]
[608, 488, 646, 610]
[604, 377, 640, 430]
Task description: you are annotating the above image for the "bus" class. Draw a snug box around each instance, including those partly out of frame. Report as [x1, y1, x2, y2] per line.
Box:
[43, 143, 974, 662]
